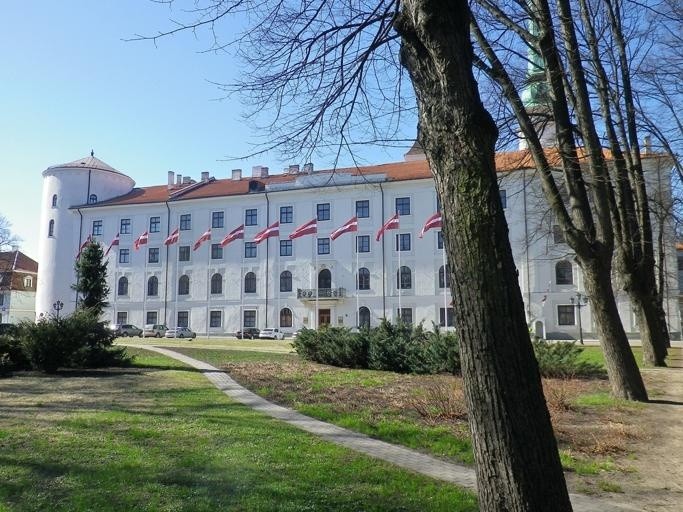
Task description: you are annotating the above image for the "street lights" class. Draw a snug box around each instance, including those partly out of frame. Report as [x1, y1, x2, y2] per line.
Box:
[52, 300, 63, 318]
[568, 291, 589, 345]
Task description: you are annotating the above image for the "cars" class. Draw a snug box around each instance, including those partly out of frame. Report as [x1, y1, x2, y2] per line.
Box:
[236, 328, 260, 340]
[166, 327, 197, 338]
[259, 328, 284, 340]
[0, 322, 18, 336]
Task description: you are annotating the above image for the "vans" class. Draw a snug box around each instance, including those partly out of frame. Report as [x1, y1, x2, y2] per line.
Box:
[143, 324, 169, 337]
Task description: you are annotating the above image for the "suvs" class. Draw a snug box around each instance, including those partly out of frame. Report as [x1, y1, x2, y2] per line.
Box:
[112, 324, 142, 336]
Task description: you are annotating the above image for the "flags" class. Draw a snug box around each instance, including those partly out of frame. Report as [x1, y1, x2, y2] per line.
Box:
[76, 237, 89, 259]
[163, 228, 179, 245]
[134, 230, 147, 250]
[418, 212, 442, 239]
[253, 221, 279, 243]
[330, 216, 357, 240]
[105, 233, 119, 256]
[192, 228, 211, 251]
[220, 224, 244, 248]
[376, 214, 399, 241]
[289, 218, 317, 239]
